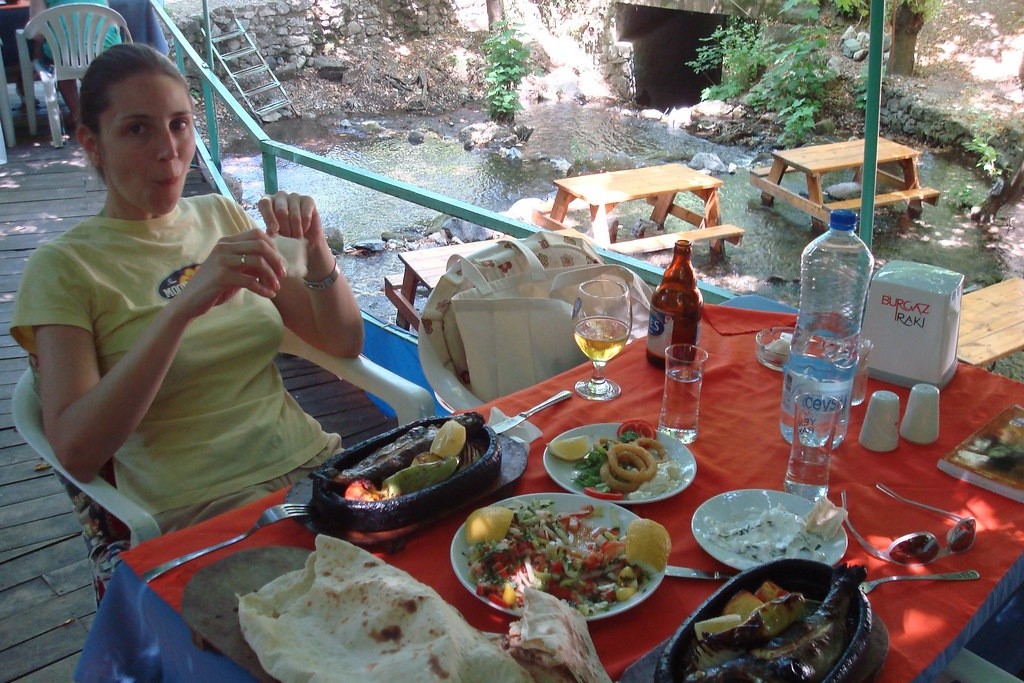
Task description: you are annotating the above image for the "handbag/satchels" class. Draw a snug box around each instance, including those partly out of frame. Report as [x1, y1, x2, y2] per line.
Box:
[446, 236, 654, 403]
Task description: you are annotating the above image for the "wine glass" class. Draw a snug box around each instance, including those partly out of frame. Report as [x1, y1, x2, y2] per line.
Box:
[570, 278, 633, 401]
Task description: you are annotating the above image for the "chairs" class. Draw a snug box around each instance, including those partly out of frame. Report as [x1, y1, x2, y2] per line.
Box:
[10, 324, 437, 547]
[418, 320, 487, 413]
[0, 38, 16, 166]
[15, 2, 134, 150]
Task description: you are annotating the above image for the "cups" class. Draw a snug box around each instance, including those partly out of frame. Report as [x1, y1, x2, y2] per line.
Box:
[785, 391, 843, 502]
[658, 344, 708, 445]
[849, 335, 873, 404]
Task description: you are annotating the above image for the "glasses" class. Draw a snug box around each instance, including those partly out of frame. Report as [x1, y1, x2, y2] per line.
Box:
[841, 480, 980, 565]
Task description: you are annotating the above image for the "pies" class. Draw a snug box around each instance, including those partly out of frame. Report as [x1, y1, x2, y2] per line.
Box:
[236, 535, 540, 683]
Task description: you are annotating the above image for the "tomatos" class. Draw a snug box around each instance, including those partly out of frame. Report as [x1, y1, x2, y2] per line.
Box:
[585, 487, 623, 499]
[616, 420, 656, 450]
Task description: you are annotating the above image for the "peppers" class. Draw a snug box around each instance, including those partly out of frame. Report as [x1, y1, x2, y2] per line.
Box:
[476, 506, 631, 610]
[370, 456, 459, 500]
[701, 591, 823, 650]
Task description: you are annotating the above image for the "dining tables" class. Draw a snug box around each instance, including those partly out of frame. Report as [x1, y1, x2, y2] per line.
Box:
[68, 294, 1024, 683]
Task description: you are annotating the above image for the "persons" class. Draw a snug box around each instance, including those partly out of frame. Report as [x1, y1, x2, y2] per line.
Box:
[0, 0, 122, 130]
[8, 43, 364, 535]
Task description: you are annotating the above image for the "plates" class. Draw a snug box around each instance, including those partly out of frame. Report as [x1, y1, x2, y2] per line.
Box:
[285, 435, 528, 546]
[614, 604, 888, 682]
[451, 494, 668, 621]
[181, 546, 311, 683]
[692, 489, 847, 572]
[543, 423, 697, 504]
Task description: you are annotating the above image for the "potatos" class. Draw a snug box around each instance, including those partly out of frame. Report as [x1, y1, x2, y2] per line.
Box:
[722, 580, 783, 622]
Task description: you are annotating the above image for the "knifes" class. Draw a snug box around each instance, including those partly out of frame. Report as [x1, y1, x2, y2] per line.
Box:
[663, 564, 734, 580]
[490, 391, 572, 434]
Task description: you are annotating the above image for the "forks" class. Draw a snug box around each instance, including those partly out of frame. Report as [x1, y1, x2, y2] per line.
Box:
[141, 504, 310, 583]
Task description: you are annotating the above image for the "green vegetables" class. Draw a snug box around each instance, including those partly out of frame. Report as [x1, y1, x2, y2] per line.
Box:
[575, 430, 641, 493]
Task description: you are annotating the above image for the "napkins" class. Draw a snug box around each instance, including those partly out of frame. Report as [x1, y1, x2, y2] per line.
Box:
[488, 406, 544, 456]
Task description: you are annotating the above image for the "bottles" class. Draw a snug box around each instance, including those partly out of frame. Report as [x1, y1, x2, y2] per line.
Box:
[859, 390, 899, 451]
[900, 385, 939, 444]
[646, 239, 704, 366]
[780, 209, 873, 448]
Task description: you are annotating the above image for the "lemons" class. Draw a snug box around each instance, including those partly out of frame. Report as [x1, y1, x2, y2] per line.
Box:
[430, 420, 466, 458]
[625, 517, 671, 573]
[693, 614, 743, 639]
[466, 507, 514, 543]
[545, 436, 593, 461]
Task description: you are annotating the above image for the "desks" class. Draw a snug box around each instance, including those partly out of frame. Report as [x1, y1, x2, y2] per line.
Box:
[760, 137, 924, 235]
[549, 162, 727, 261]
[0, 0, 170, 84]
[955, 277, 1024, 368]
[395, 239, 500, 331]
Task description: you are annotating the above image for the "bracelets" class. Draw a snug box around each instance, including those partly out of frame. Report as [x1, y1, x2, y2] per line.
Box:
[302, 256, 340, 290]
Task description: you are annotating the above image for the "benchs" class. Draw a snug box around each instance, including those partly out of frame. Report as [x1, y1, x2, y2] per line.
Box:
[821, 185, 941, 227]
[609, 223, 746, 256]
[531, 198, 590, 227]
[748, 165, 799, 186]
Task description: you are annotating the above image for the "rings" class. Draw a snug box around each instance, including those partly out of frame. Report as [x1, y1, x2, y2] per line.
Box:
[239, 254, 247, 271]
